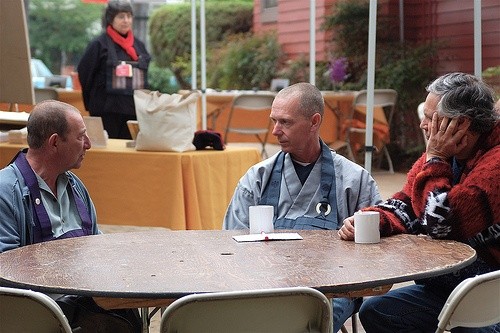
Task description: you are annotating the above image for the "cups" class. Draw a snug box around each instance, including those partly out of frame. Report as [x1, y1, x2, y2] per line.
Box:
[354, 211, 381, 244]
[249, 205, 274, 233]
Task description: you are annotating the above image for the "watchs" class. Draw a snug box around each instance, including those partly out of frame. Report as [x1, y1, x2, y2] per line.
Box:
[424, 157, 443, 164]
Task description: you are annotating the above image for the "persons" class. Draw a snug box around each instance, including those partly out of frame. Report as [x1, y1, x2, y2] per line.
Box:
[338, 70, 500, 333]
[0, 99, 145, 333]
[222, 85, 383, 333]
[77, 0, 152, 140]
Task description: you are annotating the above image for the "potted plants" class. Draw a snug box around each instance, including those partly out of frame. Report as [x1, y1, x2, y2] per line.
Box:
[375, 44, 437, 155]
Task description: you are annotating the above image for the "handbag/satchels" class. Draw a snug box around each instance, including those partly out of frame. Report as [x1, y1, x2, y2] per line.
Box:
[133, 88, 202, 152]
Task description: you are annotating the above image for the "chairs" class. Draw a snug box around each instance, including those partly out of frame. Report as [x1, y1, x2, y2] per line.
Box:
[224, 93, 275, 158]
[160, 286, 330, 333]
[0, 287, 72, 333]
[9, 88, 59, 112]
[435, 270, 500, 333]
[346, 89, 397, 175]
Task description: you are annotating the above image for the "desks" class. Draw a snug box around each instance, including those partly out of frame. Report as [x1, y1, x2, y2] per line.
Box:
[198, 89, 391, 164]
[0, 110, 28, 126]
[0, 139, 261, 230]
[0, 229, 478, 310]
[0, 90, 90, 116]
